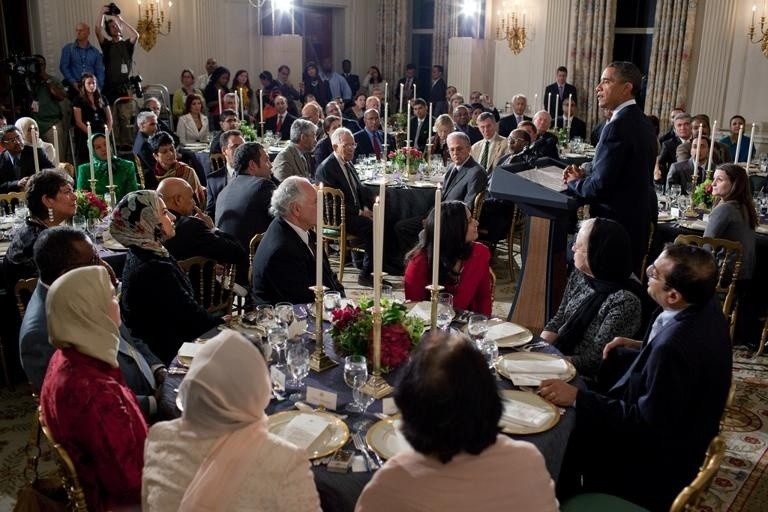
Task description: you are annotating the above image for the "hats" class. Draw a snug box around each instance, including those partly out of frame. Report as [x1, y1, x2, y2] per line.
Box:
[104, 3, 120, 15]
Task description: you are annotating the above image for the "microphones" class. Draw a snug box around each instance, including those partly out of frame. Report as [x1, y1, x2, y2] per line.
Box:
[517, 139, 548, 157]
[545, 137, 556, 149]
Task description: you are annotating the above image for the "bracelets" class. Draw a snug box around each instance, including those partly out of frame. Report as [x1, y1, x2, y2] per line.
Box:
[45, 78, 54, 85]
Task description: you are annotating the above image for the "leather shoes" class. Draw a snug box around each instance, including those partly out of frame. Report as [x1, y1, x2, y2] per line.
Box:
[358, 274, 392, 287]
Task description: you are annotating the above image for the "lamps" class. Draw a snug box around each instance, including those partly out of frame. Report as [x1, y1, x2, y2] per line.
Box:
[749, 4, 768, 56]
[496, 1, 526, 55]
[136, 0, 172, 51]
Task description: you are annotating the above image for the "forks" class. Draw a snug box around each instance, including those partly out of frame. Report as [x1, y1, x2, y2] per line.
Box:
[351, 434, 379, 470]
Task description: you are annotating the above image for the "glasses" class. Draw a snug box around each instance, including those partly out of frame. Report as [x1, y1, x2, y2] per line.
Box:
[572, 242, 587, 254]
[506, 137, 526, 143]
[646, 264, 672, 290]
[225, 118, 237, 123]
[449, 147, 467, 152]
[69, 253, 99, 266]
[28, 127, 39, 132]
[563, 102, 575, 106]
[338, 143, 357, 149]
[4, 136, 24, 143]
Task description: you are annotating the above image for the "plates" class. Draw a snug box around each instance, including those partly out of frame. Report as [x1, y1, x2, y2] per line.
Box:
[307, 299, 356, 322]
[755, 223, 768, 235]
[406, 180, 436, 188]
[363, 180, 397, 186]
[204, 149, 211, 153]
[495, 350, 577, 387]
[566, 153, 587, 159]
[266, 410, 350, 460]
[461, 320, 534, 347]
[497, 389, 561, 434]
[658, 216, 676, 221]
[175, 394, 183, 413]
[679, 220, 707, 230]
[366, 415, 415, 459]
[176, 337, 210, 367]
[181, 147, 206, 150]
[229, 311, 308, 340]
[184, 143, 208, 147]
[402, 299, 457, 331]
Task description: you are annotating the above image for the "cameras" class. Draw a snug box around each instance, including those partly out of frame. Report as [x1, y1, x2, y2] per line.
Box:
[104, 3, 121, 17]
[129, 75, 144, 98]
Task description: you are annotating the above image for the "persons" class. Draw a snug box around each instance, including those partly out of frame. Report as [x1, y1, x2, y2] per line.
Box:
[208, 109, 255, 172]
[659, 107, 686, 149]
[448, 93, 465, 117]
[250, 173, 346, 313]
[0, 124, 56, 216]
[344, 92, 368, 129]
[388, 130, 488, 276]
[204, 129, 246, 220]
[145, 98, 182, 147]
[107, 188, 233, 367]
[590, 106, 613, 148]
[314, 126, 395, 290]
[258, 94, 299, 140]
[38, 264, 150, 511]
[367, 87, 391, 117]
[353, 326, 562, 511]
[213, 141, 279, 313]
[76, 131, 139, 209]
[202, 65, 232, 131]
[15, 116, 60, 169]
[140, 328, 322, 511]
[464, 103, 472, 112]
[26, 52, 66, 165]
[358, 96, 384, 132]
[550, 97, 587, 143]
[362, 64, 390, 103]
[175, 93, 211, 151]
[72, 72, 114, 165]
[538, 215, 643, 392]
[394, 63, 420, 113]
[156, 177, 244, 316]
[312, 114, 360, 171]
[255, 67, 281, 116]
[271, 119, 318, 188]
[716, 115, 757, 165]
[647, 113, 660, 137]
[543, 66, 578, 121]
[531, 108, 559, 148]
[429, 64, 445, 109]
[475, 129, 538, 241]
[517, 120, 560, 161]
[145, 129, 205, 210]
[470, 111, 512, 177]
[469, 102, 485, 127]
[538, 242, 734, 511]
[432, 85, 458, 118]
[676, 113, 732, 169]
[304, 93, 314, 104]
[297, 103, 325, 143]
[698, 163, 757, 300]
[658, 111, 694, 192]
[17, 225, 167, 430]
[207, 93, 252, 133]
[470, 90, 501, 123]
[94, 5, 141, 114]
[400, 96, 440, 154]
[229, 67, 258, 117]
[269, 64, 307, 115]
[298, 61, 333, 119]
[2, 168, 78, 323]
[59, 22, 106, 107]
[312, 100, 325, 119]
[498, 92, 534, 138]
[331, 93, 345, 115]
[452, 105, 484, 146]
[172, 67, 208, 119]
[317, 56, 352, 101]
[0, 108, 9, 156]
[561, 60, 662, 284]
[132, 109, 199, 187]
[193, 56, 219, 91]
[665, 135, 719, 198]
[317, 100, 364, 140]
[261, 87, 282, 121]
[340, 59, 360, 98]
[423, 112, 458, 168]
[354, 109, 397, 160]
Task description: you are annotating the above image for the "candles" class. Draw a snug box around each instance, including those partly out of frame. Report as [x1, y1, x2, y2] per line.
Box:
[534, 92, 571, 127]
[218, 88, 263, 122]
[384, 83, 433, 145]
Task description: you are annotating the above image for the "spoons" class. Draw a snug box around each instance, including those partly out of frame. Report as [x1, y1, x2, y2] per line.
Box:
[295, 401, 348, 420]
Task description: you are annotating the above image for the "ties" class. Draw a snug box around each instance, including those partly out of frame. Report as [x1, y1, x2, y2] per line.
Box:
[441, 167, 458, 197]
[372, 134, 382, 157]
[414, 121, 422, 146]
[308, 234, 317, 256]
[559, 86, 563, 97]
[655, 318, 663, 334]
[516, 116, 521, 124]
[300, 157, 308, 171]
[697, 166, 703, 183]
[344, 163, 357, 199]
[406, 78, 412, 92]
[276, 116, 283, 132]
[480, 141, 489, 169]
[14, 156, 21, 179]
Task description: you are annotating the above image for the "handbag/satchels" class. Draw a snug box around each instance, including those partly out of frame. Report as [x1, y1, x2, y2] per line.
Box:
[12, 408, 76, 512]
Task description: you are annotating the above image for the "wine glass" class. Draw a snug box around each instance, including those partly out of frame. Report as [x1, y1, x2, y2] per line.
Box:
[657, 183, 681, 208]
[283, 337, 305, 390]
[265, 128, 283, 147]
[207, 133, 214, 147]
[571, 136, 584, 154]
[324, 290, 342, 335]
[418, 152, 447, 181]
[356, 152, 377, 179]
[343, 355, 368, 413]
[286, 347, 310, 403]
[677, 196, 691, 224]
[274, 302, 294, 340]
[476, 340, 500, 383]
[255, 303, 274, 344]
[0, 200, 31, 242]
[437, 293, 456, 318]
[86, 217, 99, 246]
[103, 192, 117, 207]
[349, 374, 376, 433]
[751, 151, 768, 172]
[73, 215, 86, 233]
[467, 314, 490, 350]
[212, 131, 218, 139]
[266, 320, 287, 373]
[436, 303, 453, 334]
[753, 190, 768, 216]
[380, 284, 392, 301]
